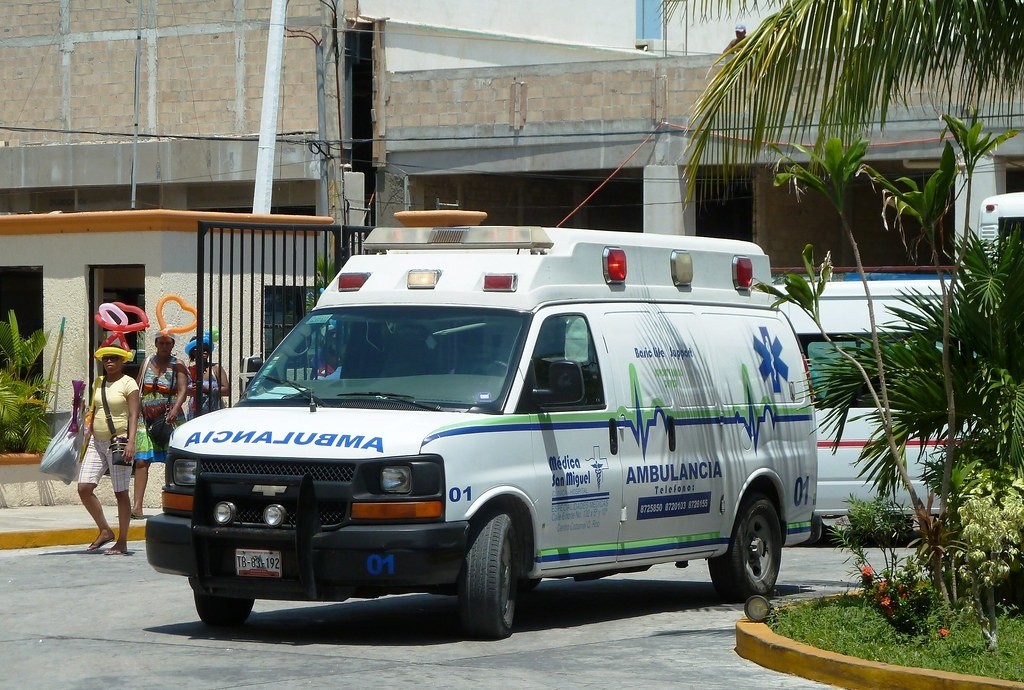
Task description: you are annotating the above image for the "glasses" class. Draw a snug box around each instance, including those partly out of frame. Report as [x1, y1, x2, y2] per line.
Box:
[101, 355, 122, 361]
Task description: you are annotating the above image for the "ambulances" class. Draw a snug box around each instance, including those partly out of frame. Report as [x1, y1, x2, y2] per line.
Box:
[146, 207, 817, 640]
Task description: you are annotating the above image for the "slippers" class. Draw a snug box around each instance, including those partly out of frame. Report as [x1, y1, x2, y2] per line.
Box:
[103, 546, 128, 555]
[86, 537, 115, 552]
[130, 510, 145, 519]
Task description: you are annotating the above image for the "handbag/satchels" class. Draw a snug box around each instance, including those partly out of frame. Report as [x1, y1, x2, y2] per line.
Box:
[110, 437, 132, 465]
[40, 408, 85, 486]
[147, 413, 176, 446]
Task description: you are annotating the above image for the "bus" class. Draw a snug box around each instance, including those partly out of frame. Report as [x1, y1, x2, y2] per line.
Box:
[978, 192, 1023, 270]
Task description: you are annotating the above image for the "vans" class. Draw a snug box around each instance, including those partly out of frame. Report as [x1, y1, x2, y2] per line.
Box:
[770, 273, 988, 539]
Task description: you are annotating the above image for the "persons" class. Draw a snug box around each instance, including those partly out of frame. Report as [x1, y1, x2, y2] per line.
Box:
[722, 24, 751, 53]
[128, 327, 189, 520]
[71, 345, 141, 556]
[184, 336, 231, 421]
